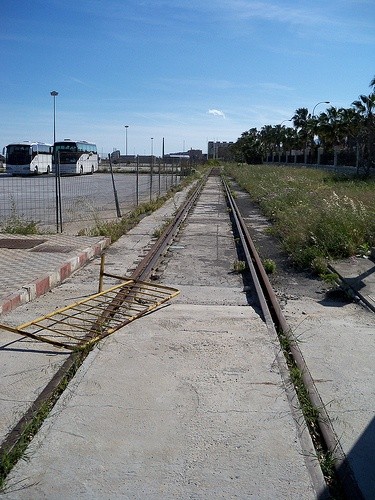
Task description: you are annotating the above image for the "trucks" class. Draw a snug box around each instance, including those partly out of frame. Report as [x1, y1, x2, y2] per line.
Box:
[111, 151, 136, 165]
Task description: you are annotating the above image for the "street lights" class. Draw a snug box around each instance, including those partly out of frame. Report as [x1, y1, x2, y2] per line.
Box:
[150, 138, 154, 156]
[125, 125, 129, 156]
[51, 91, 59, 144]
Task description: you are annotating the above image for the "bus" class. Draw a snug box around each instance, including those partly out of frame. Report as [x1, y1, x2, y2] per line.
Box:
[49, 139, 99, 176]
[3, 140, 53, 177]
